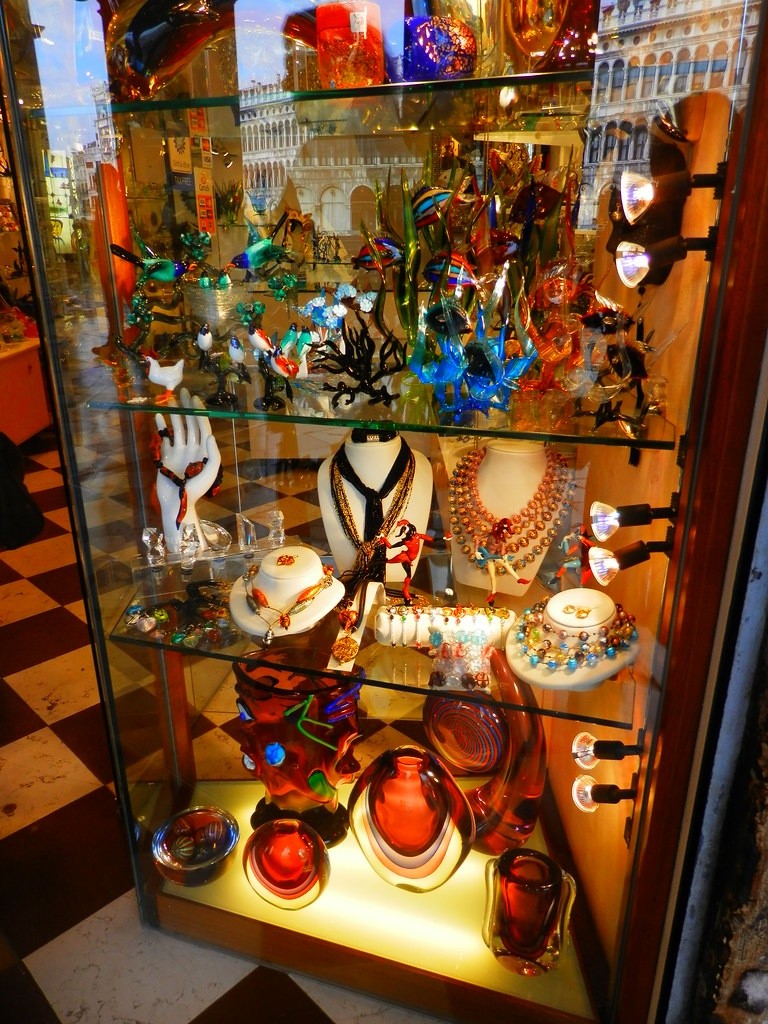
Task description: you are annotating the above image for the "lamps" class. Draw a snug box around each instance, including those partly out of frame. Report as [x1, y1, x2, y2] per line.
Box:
[571, 727, 645, 771]
[620, 161, 726, 226]
[588, 526, 676, 586]
[615, 225, 718, 289]
[589, 491, 680, 543]
[571, 773, 640, 813]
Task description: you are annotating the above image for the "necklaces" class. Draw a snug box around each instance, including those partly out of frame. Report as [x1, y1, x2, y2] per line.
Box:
[449, 449, 577, 575]
[331, 438, 416, 607]
[153, 452, 224, 530]
[332, 579, 372, 663]
[515, 597, 638, 669]
[243, 563, 334, 645]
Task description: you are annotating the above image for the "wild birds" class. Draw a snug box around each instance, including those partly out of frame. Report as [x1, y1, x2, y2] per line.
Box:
[245, 320, 312, 404]
[226, 329, 247, 374]
[198, 266, 212, 289]
[110, 226, 197, 282]
[224, 211, 297, 275]
[212, 267, 233, 290]
[197, 322, 212, 356]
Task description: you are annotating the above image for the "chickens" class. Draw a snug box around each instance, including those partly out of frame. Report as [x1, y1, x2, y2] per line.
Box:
[138, 346, 185, 406]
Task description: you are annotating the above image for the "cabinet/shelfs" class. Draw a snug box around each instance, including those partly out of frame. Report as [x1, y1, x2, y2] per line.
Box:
[0, 336, 54, 448]
[0, 0, 768, 1023]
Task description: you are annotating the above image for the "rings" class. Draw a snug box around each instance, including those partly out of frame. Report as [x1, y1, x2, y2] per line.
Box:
[159, 428, 169, 437]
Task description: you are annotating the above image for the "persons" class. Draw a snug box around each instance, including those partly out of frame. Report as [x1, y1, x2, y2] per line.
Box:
[228, 4, 448, 596]
[153, 389, 221, 554]
[91, 164, 142, 358]
[376, 519, 452, 607]
[474, 518, 530, 602]
